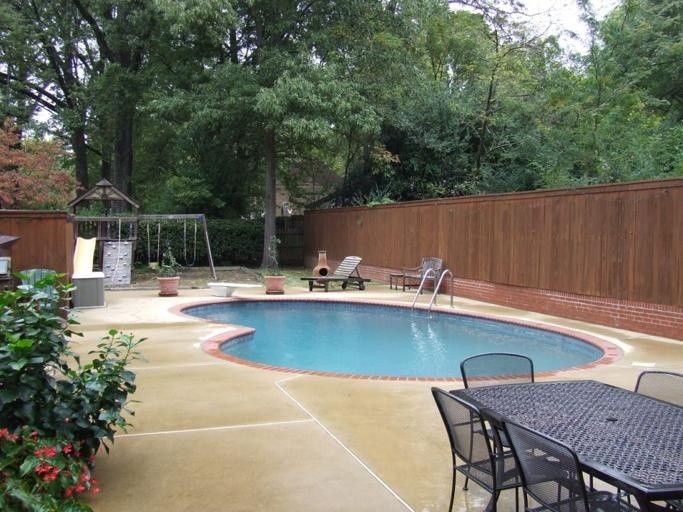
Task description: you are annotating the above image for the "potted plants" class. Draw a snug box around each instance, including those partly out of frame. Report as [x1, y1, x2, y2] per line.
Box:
[154, 242, 182, 296]
[263, 233, 287, 294]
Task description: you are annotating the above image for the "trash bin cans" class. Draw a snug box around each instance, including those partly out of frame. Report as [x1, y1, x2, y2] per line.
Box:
[313, 250, 332, 288]
[71, 271, 105, 307]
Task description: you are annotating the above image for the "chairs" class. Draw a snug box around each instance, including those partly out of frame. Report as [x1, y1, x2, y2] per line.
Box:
[20, 269, 56, 311]
[300, 255, 372, 293]
[389, 257, 444, 295]
